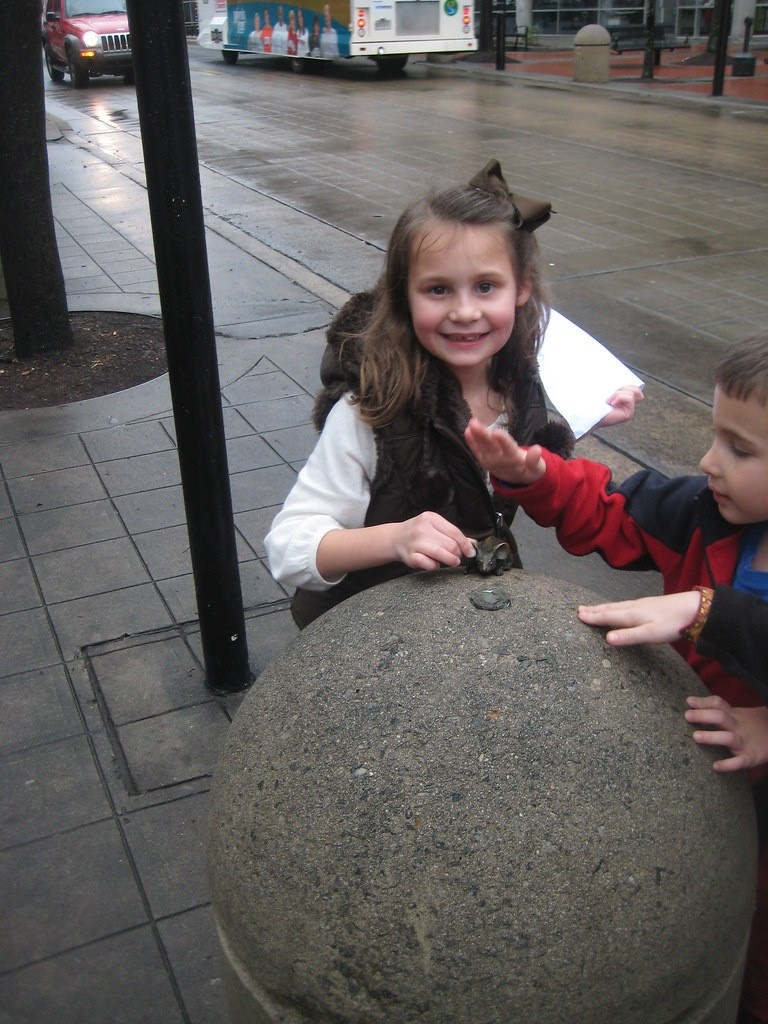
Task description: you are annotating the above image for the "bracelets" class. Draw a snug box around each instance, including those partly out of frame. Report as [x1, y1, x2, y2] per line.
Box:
[679, 585, 714, 641]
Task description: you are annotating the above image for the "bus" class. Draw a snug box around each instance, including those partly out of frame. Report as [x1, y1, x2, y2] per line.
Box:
[199, 1, 482, 72]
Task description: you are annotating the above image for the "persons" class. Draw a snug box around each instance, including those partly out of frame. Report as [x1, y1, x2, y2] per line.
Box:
[263, 157, 645, 630]
[248, 4, 340, 58]
[464, 334, 768, 1024]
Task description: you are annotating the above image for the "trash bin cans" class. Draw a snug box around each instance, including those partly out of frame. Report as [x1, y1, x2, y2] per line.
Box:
[203, 562, 768, 1024]
[573, 24, 612, 84]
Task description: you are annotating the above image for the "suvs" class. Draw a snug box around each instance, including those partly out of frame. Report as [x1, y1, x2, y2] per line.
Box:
[42, 1, 137, 91]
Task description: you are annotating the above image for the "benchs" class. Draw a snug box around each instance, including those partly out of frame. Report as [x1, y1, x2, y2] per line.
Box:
[607, 23, 692, 54]
[492, 25, 529, 51]
